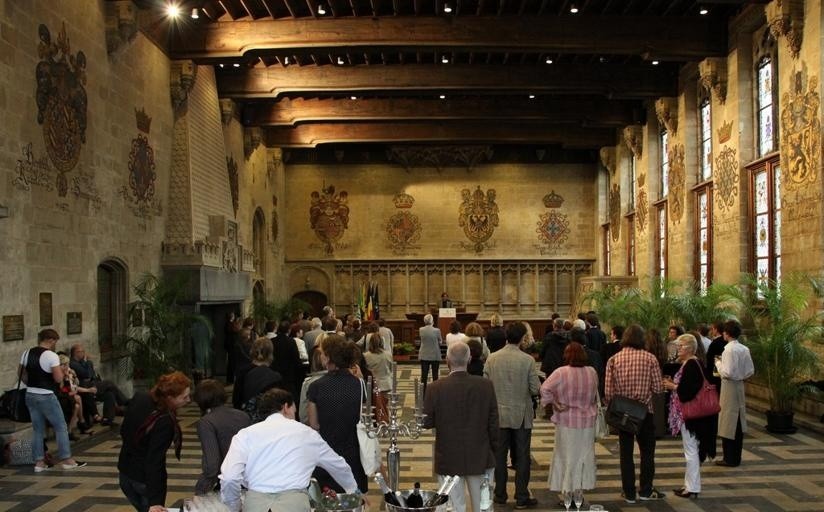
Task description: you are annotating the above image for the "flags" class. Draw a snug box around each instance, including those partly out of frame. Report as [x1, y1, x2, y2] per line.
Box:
[350, 279, 382, 323]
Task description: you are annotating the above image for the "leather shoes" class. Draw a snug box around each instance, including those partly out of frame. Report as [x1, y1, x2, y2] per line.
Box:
[715, 460, 729, 464]
[493, 496, 505, 504]
[516, 498, 537, 509]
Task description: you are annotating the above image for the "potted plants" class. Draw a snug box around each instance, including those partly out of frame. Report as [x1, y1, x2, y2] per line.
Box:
[524, 340, 543, 362]
[393, 342, 415, 365]
[98, 270, 215, 412]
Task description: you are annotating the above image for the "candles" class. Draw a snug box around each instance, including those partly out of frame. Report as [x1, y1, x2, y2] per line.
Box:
[366, 361, 425, 415]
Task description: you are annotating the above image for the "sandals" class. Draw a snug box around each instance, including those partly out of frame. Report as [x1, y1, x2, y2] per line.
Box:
[68, 415, 112, 440]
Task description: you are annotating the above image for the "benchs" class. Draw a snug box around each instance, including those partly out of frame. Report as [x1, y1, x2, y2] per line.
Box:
[0, 401, 104, 465]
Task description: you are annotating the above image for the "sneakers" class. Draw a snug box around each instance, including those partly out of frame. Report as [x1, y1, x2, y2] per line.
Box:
[621, 489, 665, 504]
[63, 461, 87, 469]
[34, 463, 54, 472]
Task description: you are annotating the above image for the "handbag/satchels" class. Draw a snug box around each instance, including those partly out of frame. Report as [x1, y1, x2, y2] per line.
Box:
[606, 396, 648, 433]
[0, 387, 33, 423]
[596, 402, 609, 440]
[356, 423, 381, 477]
[677, 379, 721, 419]
[372, 388, 389, 424]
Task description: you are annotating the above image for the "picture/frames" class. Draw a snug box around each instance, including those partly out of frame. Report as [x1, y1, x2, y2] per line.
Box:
[66, 312, 83, 335]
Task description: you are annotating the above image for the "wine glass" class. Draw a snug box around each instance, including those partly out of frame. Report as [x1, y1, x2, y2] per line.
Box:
[562, 485, 584, 512]
[662, 375, 670, 393]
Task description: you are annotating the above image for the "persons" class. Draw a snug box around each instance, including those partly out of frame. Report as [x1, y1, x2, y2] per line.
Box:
[544, 311, 667, 376]
[304, 333, 369, 494]
[188, 379, 252, 497]
[482, 321, 542, 509]
[418, 314, 491, 403]
[714, 320, 755, 467]
[537, 341, 599, 509]
[421, 342, 503, 512]
[602, 323, 667, 504]
[116, 370, 193, 511]
[7, 324, 131, 471]
[667, 321, 726, 386]
[435, 292, 453, 311]
[224, 306, 341, 421]
[342, 313, 394, 404]
[662, 333, 719, 499]
[217, 386, 372, 512]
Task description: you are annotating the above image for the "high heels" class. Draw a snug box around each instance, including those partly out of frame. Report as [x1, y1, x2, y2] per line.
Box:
[676, 488, 698, 499]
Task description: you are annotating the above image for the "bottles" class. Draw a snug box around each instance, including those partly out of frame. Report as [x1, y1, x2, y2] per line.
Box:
[371, 376, 388, 424]
[375, 472, 459, 506]
[322, 486, 362, 509]
[712, 355, 722, 377]
[478, 472, 494, 512]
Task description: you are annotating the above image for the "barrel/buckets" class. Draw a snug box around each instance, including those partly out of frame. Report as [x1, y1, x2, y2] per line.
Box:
[319, 494, 364, 512]
[385, 488, 450, 512]
